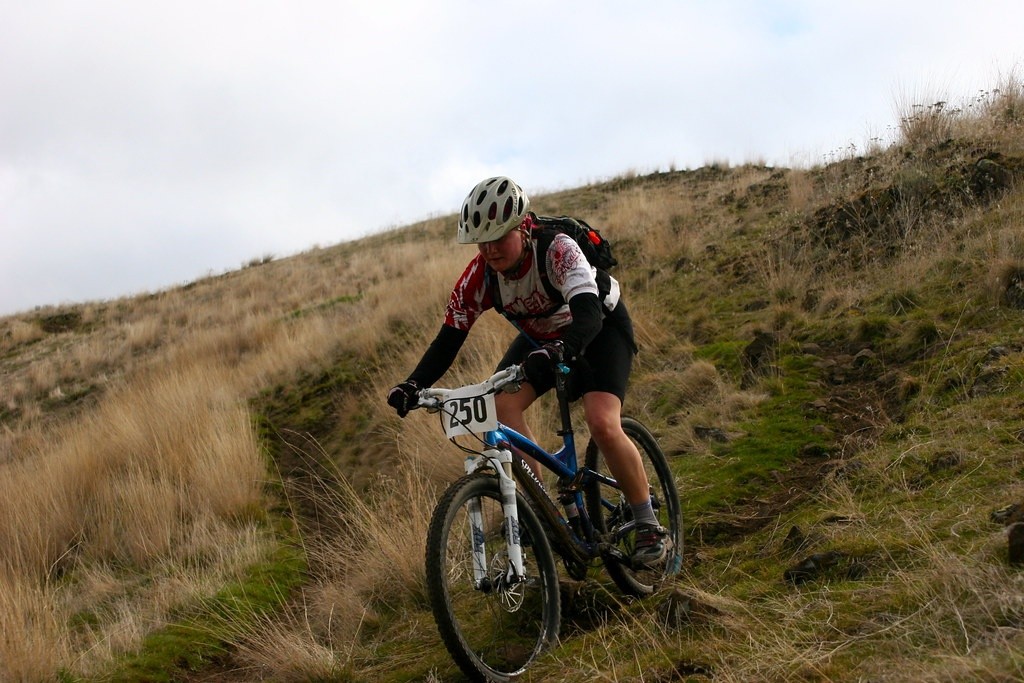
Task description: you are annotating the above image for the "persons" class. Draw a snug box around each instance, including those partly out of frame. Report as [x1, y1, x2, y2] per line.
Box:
[386, 176, 673, 566]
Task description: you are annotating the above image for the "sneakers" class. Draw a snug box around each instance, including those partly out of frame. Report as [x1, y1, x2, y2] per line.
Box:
[631, 522, 674, 566]
[501, 520, 530, 546]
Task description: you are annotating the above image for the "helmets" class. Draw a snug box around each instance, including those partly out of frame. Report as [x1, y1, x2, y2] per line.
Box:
[458, 177, 530, 245]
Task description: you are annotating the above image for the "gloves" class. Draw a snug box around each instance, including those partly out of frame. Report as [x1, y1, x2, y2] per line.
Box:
[388, 380, 421, 419]
[525, 346, 557, 387]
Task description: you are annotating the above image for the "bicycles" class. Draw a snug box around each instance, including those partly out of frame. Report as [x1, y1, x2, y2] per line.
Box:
[387, 339, 685, 682]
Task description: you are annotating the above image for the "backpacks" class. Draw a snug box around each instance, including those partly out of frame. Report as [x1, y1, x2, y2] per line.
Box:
[486, 212, 616, 314]
